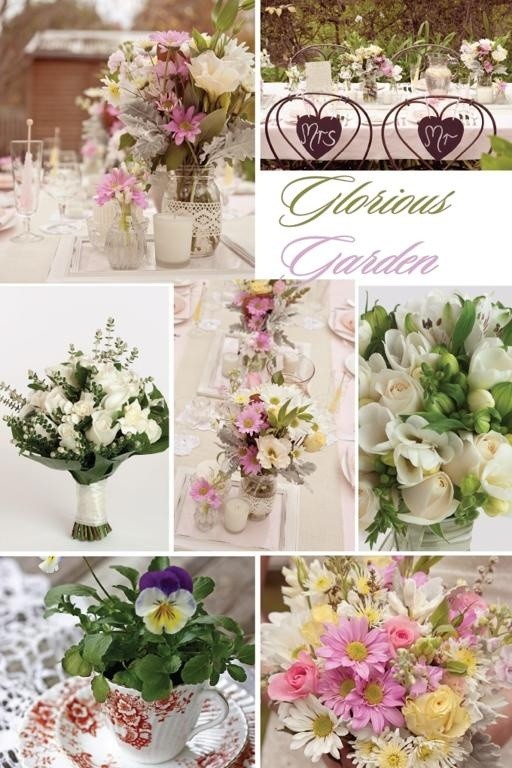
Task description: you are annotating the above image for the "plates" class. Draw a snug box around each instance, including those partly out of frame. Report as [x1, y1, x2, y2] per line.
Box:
[328, 310, 354, 341]
[16, 669, 254, 768]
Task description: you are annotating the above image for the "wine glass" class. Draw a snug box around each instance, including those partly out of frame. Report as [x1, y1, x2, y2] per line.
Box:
[40, 148, 84, 235]
[10, 140, 44, 244]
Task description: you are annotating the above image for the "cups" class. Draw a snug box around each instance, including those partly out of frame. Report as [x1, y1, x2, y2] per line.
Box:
[266, 352, 317, 399]
[93, 670, 229, 763]
[424, 52, 460, 98]
[458, 76, 470, 96]
[192, 396, 211, 423]
